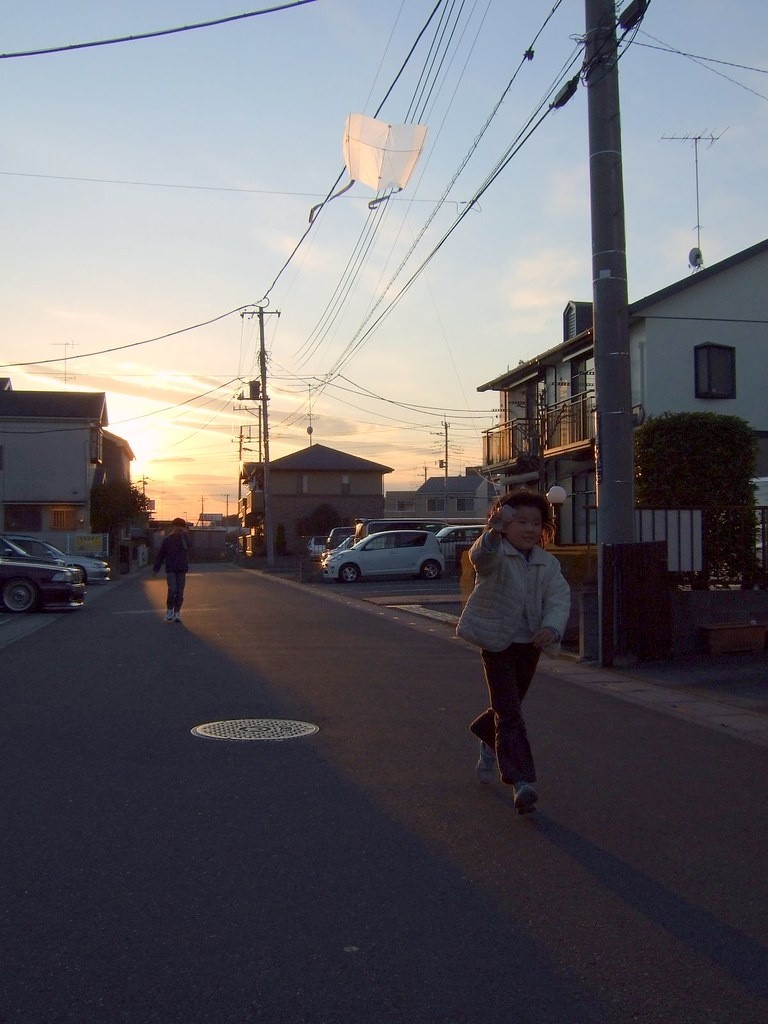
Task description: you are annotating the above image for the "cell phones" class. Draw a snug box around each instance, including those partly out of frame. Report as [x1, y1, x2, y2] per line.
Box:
[489, 504, 517, 531]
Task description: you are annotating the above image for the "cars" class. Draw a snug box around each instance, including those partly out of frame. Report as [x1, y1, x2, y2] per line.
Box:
[0, 534, 86, 615]
[2, 534, 112, 587]
[320, 529, 446, 585]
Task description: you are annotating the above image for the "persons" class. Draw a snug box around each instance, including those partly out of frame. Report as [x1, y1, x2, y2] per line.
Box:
[456, 490, 571, 812]
[151, 518, 193, 623]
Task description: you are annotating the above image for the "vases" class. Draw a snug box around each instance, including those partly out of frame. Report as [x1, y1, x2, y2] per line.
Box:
[698, 621, 768, 659]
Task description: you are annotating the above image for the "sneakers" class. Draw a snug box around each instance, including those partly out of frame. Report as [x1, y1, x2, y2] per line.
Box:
[513, 784, 538, 815]
[166, 609, 173, 621]
[174, 614, 181, 622]
[475, 741, 496, 784]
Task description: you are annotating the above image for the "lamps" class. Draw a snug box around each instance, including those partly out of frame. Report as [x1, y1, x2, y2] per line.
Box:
[548, 486, 566, 507]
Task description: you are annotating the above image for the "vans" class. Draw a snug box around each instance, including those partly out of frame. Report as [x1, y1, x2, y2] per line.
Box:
[304, 517, 493, 570]
